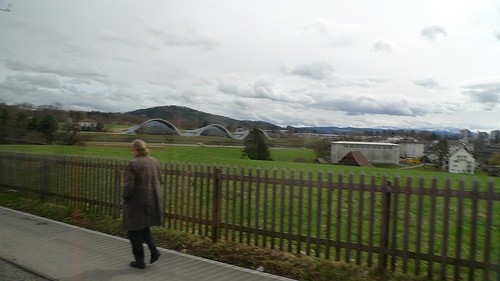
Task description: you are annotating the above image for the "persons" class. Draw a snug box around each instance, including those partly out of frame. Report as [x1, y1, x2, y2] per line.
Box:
[121, 139, 164, 269]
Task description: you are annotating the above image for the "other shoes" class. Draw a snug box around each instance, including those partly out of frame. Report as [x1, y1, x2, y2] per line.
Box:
[150, 250, 161, 264]
[130, 261, 145, 269]
